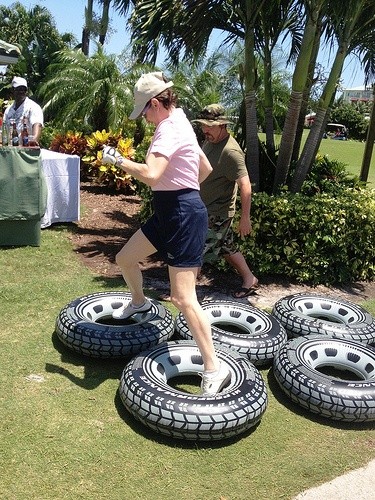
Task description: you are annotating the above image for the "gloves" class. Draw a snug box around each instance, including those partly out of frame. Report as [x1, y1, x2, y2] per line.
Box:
[100, 144, 124, 166]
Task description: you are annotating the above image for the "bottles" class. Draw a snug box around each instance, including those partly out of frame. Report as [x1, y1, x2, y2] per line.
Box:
[0, 130, 3, 143]
[10, 119, 19, 146]
[22, 116, 29, 146]
[18, 121, 23, 146]
[8, 126, 13, 146]
[2, 120, 8, 146]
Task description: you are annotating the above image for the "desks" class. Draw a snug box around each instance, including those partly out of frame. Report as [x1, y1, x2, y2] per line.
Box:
[40, 147, 80, 229]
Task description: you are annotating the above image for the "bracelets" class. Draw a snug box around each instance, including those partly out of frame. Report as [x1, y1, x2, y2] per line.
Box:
[116, 156, 125, 167]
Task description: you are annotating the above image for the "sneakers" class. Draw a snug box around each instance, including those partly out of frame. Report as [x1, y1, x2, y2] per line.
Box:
[198, 362, 232, 395]
[112, 300, 153, 320]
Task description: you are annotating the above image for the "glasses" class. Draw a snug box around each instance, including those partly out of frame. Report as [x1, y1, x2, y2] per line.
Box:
[14, 90, 26, 95]
[142, 104, 150, 118]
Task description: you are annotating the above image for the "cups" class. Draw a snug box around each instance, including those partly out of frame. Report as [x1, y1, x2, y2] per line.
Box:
[28, 135, 36, 146]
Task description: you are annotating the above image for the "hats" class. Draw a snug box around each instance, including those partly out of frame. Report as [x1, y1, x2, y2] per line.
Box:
[129, 72, 174, 119]
[12, 77, 27, 88]
[191, 104, 235, 127]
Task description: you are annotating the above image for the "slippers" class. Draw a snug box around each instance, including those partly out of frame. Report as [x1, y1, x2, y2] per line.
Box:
[233, 285, 260, 298]
[158, 289, 172, 301]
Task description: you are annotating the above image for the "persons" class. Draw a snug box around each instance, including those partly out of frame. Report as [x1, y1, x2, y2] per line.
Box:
[3, 77, 44, 143]
[97, 71, 231, 398]
[157, 103, 258, 302]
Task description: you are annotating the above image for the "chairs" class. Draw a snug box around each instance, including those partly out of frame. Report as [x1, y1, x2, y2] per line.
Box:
[0, 147, 48, 246]
